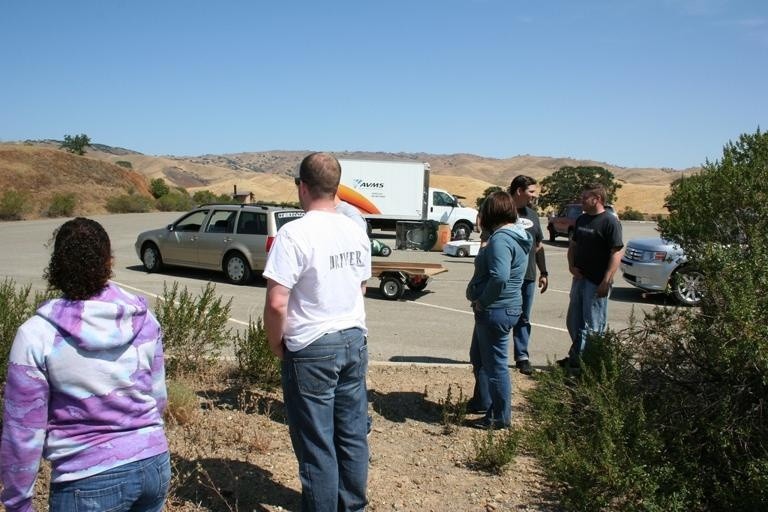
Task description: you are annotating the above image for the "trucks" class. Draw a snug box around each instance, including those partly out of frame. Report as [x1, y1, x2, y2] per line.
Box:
[338, 160, 479, 240]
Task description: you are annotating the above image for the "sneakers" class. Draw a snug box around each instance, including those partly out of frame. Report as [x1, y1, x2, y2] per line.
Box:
[516, 357, 532, 375]
[459, 397, 488, 414]
[469, 412, 511, 430]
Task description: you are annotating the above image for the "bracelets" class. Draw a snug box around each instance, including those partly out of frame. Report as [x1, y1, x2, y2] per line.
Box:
[540, 270, 548, 276]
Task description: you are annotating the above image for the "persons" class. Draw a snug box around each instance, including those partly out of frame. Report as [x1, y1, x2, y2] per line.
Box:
[479, 172, 551, 377]
[260, 152, 373, 511]
[476, 191, 497, 235]
[0, 215, 172, 510]
[455, 189, 534, 430]
[332, 191, 374, 438]
[554, 182, 626, 368]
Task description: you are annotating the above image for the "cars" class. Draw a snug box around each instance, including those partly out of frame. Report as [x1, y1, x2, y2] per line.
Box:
[548, 203, 619, 241]
[619, 238, 707, 306]
[135, 203, 305, 285]
[444, 240, 481, 256]
